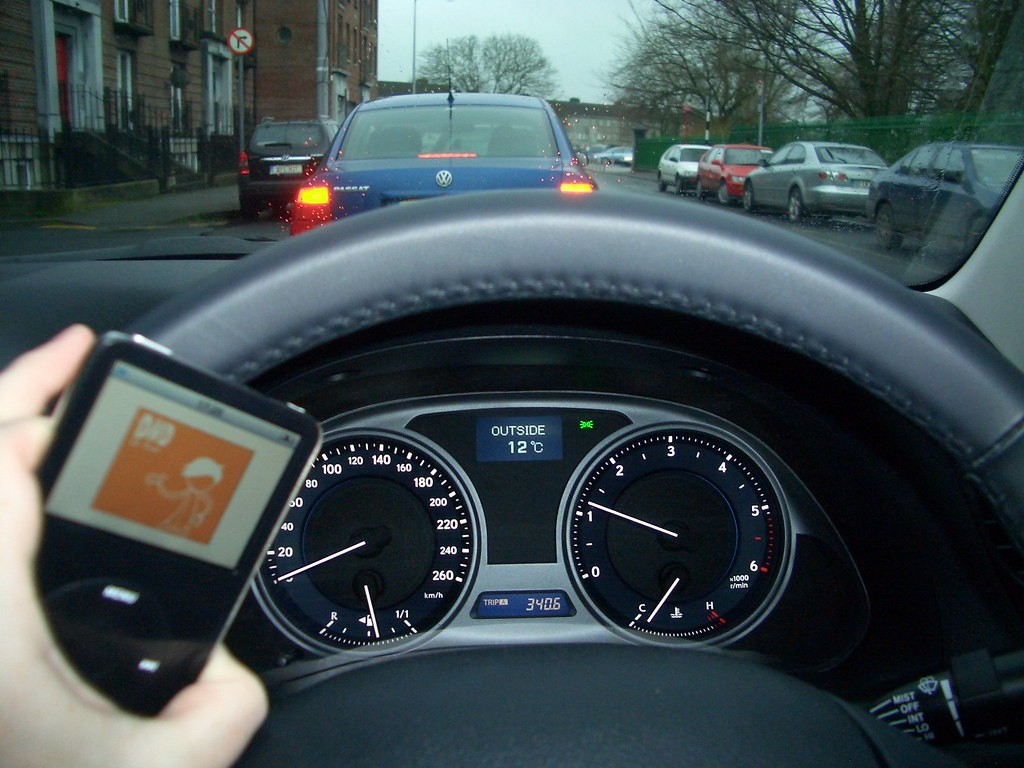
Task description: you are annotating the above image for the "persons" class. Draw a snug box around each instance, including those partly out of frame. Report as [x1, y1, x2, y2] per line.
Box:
[1, 323, 269, 768]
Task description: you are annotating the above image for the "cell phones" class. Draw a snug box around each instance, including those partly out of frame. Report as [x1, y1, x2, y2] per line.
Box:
[32, 329, 324, 720]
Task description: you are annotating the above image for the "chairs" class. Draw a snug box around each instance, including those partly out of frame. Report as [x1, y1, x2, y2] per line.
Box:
[366, 126, 423, 159]
[487, 129, 538, 157]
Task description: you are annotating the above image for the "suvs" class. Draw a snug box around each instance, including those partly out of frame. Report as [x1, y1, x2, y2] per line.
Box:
[239, 114, 343, 222]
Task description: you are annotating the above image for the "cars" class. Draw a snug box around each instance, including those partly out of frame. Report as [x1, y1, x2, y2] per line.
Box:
[864, 143, 1024, 262]
[741, 140, 888, 223]
[697, 143, 778, 207]
[592, 145, 634, 168]
[289, 91, 599, 237]
[657, 143, 715, 196]
